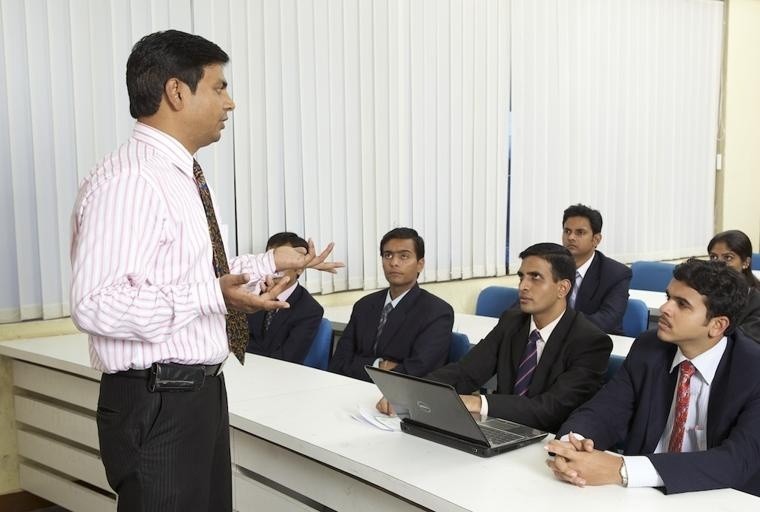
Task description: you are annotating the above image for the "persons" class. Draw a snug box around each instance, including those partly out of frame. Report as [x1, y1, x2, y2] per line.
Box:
[331, 227, 454, 379]
[247, 232, 325, 365]
[374, 242, 614, 435]
[707, 230, 760, 344]
[70, 30, 346, 512]
[561, 204, 633, 336]
[543, 258, 759, 497]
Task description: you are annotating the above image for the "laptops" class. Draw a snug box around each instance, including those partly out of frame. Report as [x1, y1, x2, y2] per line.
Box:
[364, 365, 549, 458]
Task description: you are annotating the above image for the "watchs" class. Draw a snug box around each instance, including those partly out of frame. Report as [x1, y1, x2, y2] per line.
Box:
[620, 459, 629, 488]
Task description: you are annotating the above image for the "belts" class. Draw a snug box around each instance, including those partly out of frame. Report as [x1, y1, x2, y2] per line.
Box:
[129, 357, 227, 381]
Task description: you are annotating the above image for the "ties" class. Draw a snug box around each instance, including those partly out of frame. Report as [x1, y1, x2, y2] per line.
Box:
[514, 331, 543, 397]
[191, 157, 248, 366]
[568, 272, 579, 312]
[668, 359, 697, 452]
[260, 307, 276, 338]
[374, 302, 393, 359]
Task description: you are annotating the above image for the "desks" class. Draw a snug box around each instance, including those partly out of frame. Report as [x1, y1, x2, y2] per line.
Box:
[0, 334, 759, 512]
[627, 288, 668, 317]
[321, 306, 636, 385]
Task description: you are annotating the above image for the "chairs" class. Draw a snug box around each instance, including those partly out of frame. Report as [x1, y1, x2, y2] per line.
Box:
[304, 318, 335, 370]
[623, 299, 650, 339]
[629, 261, 674, 292]
[447, 332, 469, 362]
[606, 355, 625, 380]
[751, 253, 760, 270]
[476, 286, 519, 318]
